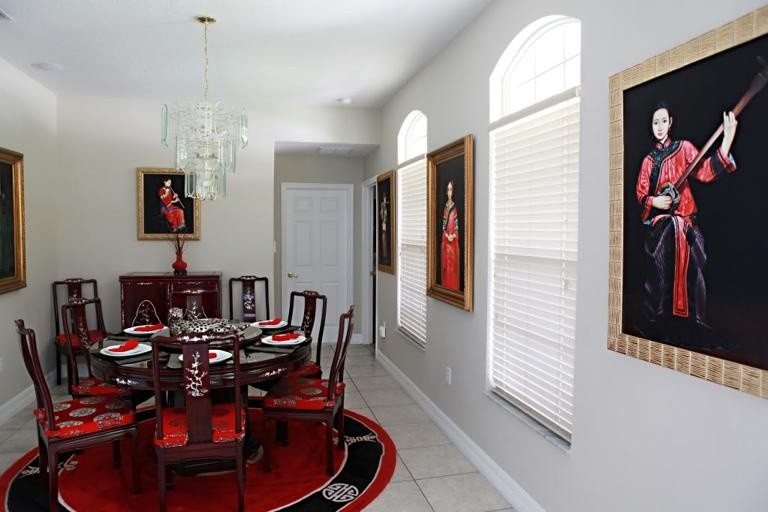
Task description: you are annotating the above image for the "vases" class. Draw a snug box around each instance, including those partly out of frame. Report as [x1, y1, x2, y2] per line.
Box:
[172, 251, 187, 275]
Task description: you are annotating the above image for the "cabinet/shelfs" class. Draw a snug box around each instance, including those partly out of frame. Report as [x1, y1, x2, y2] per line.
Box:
[119, 275, 225, 330]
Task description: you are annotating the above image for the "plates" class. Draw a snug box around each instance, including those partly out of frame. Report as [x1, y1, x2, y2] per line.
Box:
[177, 350, 237, 367]
[249, 319, 289, 330]
[122, 323, 166, 337]
[98, 343, 152, 359]
[261, 333, 307, 347]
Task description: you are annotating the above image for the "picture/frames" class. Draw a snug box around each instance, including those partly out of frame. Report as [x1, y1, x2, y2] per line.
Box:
[0, 147, 27, 295]
[136, 167, 200, 241]
[426, 134, 474, 313]
[607, 3, 768, 399]
[377, 169, 394, 274]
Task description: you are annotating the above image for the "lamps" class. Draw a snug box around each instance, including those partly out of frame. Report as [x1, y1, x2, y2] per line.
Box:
[161, 17, 247, 201]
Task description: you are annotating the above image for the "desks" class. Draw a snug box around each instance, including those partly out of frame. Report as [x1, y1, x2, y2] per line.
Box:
[84, 315, 320, 480]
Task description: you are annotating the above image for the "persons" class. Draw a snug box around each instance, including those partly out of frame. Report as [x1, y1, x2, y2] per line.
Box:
[635, 101, 740, 352]
[376, 195, 389, 264]
[159, 177, 187, 232]
[440, 179, 461, 291]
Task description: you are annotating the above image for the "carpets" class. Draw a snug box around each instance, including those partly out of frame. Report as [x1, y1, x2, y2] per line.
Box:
[1, 397, 397, 512]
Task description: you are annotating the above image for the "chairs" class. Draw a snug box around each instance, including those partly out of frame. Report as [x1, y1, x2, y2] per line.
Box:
[149, 331, 257, 511]
[257, 304, 355, 479]
[164, 283, 223, 320]
[229, 275, 272, 324]
[50, 277, 109, 382]
[59, 296, 164, 472]
[269, 288, 330, 428]
[14, 317, 144, 511]
